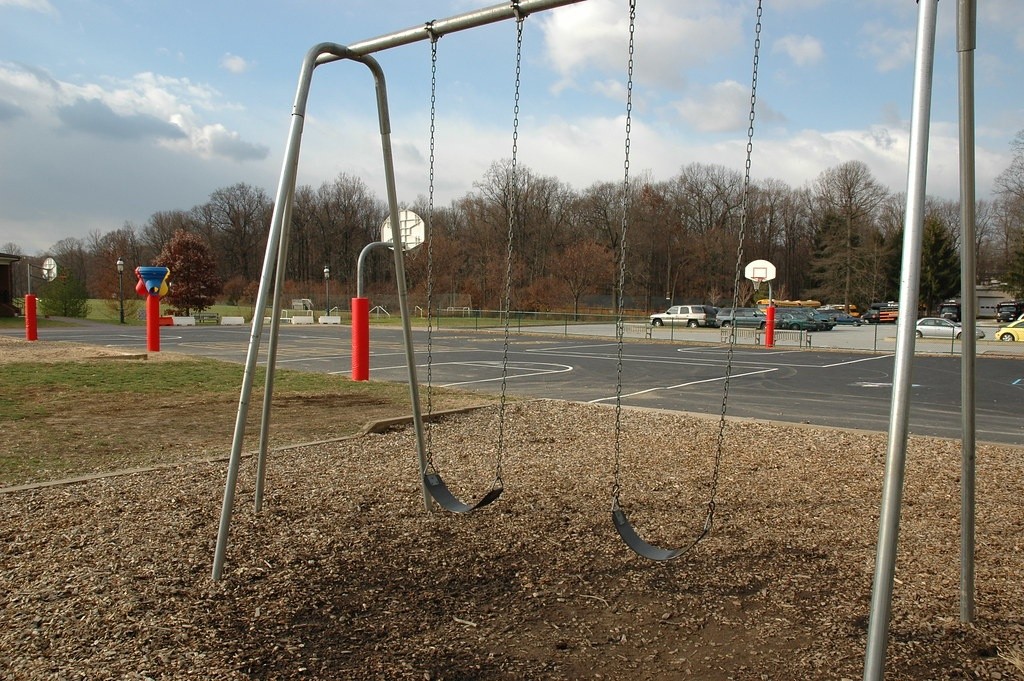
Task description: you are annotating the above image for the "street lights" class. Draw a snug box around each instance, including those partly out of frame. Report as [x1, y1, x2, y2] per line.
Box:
[322, 265, 331, 317]
[115, 257, 126, 324]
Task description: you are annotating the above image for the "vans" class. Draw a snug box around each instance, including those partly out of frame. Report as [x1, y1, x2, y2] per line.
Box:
[860, 301, 899, 326]
[831, 304, 861, 317]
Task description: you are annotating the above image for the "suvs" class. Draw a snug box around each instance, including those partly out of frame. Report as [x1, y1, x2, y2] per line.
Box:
[770, 307, 837, 332]
[649, 304, 716, 329]
[714, 306, 784, 331]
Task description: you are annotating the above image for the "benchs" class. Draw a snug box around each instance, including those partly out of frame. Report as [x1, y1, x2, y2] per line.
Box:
[720, 326, 760, 346]
[191, 313, 220, 324]
[773, 330, 812, 348]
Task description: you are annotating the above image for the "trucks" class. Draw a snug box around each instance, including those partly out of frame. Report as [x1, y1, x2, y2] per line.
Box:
[939, 296, 962, 323]
[995, 300, 1024, 323]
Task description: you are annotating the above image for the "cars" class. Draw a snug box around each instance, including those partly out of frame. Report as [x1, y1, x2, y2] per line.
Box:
[916, 316, 986, 341]
[995, 317, 1024, 343]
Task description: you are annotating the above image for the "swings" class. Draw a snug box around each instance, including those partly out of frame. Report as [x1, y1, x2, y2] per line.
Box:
[423, 18, 524, 514]
[611, 1, 763, 562]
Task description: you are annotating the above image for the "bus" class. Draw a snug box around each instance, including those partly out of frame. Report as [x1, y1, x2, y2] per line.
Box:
[756, 300, 820, 317]
